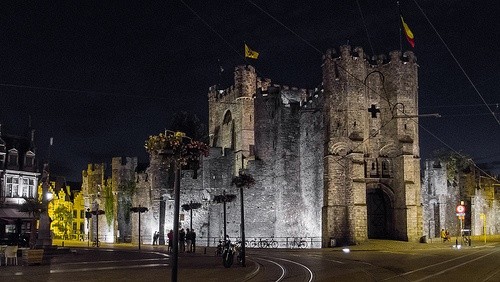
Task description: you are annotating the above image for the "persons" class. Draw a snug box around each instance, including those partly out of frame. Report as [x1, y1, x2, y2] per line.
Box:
[446, 229, 452, 242]
[150, 227, 198, 254]
[441, 229, 447, 243]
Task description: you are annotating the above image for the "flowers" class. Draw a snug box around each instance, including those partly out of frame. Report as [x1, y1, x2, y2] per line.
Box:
[143, 133, 210, 158]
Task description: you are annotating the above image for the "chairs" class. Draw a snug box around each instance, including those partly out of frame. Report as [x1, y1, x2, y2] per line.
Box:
[0, 245, 19, 266]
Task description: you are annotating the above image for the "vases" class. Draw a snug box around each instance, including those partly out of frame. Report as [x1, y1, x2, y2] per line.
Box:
[156, 149, 175, 155]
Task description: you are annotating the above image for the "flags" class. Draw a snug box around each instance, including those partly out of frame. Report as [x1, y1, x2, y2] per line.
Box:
[217, 60, 226, 76]
[244, 43, 259, 59]
[399, 12, 418, 48]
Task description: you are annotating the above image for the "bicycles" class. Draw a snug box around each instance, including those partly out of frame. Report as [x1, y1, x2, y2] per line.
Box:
[92, 238, 101, 247]
[290, 236, 307, 250]
[235, 234, 278, 249]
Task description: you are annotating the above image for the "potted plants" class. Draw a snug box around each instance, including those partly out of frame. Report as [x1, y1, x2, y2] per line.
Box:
[16, 194, 47, 265]
